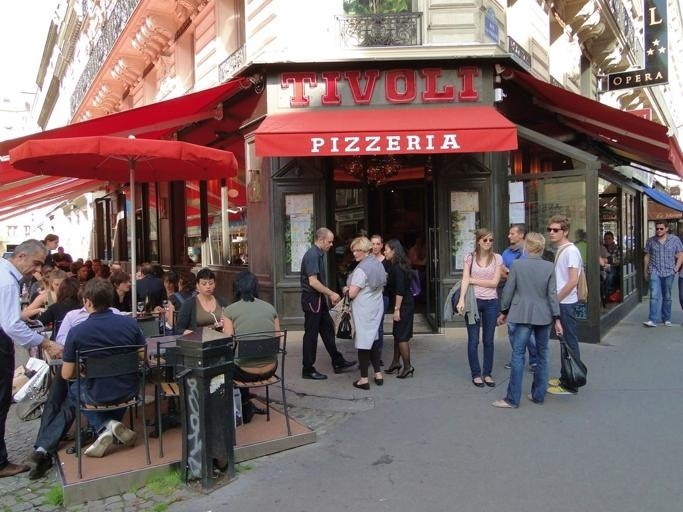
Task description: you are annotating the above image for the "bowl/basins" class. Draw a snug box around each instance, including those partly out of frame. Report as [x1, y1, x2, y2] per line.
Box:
[187, 246, 200, 263]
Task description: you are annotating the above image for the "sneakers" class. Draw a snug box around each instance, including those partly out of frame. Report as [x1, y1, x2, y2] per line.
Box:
[664, 320, 673, 327]
[643, 320, 657, 326]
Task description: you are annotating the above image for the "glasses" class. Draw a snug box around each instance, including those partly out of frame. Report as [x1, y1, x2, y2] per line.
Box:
[545, 226, 565, 232]
[656, 227, 668, 231]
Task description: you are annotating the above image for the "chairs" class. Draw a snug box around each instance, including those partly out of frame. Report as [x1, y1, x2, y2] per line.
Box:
[69, 344, 151, 479]
[156, 340, 182, 459]
[21, 299, 183, 356]
[230, 329, 293, 437]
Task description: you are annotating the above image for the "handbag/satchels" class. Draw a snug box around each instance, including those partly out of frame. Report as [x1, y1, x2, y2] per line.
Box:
[577, 270, 591, 304]
[410, 269, 422, 296]
[560, 346, 587, 388]
[335, 306, 355, 340]
[12, 358, 47, 419]
[451, 283, 470, 316]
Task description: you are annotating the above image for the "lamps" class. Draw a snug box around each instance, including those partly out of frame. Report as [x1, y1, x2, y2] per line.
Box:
[246, 169, 262, 203]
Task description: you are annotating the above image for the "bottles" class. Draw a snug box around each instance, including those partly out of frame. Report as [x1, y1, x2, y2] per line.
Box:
[21, 282, 29, 300]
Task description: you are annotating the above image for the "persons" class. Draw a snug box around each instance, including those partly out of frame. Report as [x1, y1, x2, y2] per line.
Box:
[342, 235, 387, 391]
[1, 239, 63, 478]
[455, 227, 501, 388]
[383, 238, 420, 379]
[674, 251, 682, 308]
[300, 226, 357, 380]
[574, 227, 603, 298]
[20, 234, 281, 483]
[490, 231, 562, 409]
[370, 233, 390, 366]
[599, 231, 620, 295]
[639, 219, 682, 327]
[500, 223, 541, 372]
[546, 214, 588, 395]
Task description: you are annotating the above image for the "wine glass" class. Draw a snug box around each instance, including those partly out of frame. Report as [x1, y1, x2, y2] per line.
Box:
[137, 301, 144, 318]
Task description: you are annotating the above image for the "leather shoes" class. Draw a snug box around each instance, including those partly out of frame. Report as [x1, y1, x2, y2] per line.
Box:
[0, 421, 136, 479]
[303, 359, 577, 408]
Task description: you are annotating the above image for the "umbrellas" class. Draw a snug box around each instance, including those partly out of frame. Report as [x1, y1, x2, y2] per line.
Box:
[7, 134, 237, 317]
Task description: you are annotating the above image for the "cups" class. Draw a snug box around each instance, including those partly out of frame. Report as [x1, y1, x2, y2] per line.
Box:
[162, 299, 168, 308]
